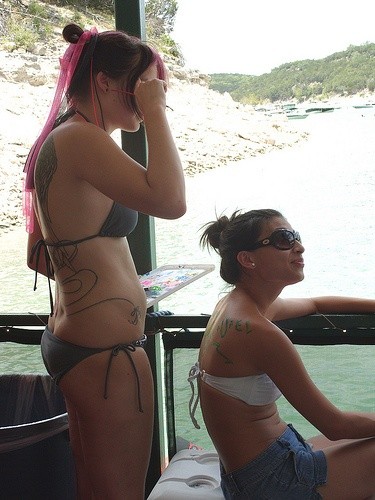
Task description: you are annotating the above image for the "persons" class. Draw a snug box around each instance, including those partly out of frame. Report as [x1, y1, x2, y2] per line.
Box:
[27, 23, 187, 500]
[197, 208, 375, 500]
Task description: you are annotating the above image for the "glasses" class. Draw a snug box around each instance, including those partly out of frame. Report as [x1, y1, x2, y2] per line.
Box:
[111, 88, 174, 117]
[253, 230, 301, 250]
